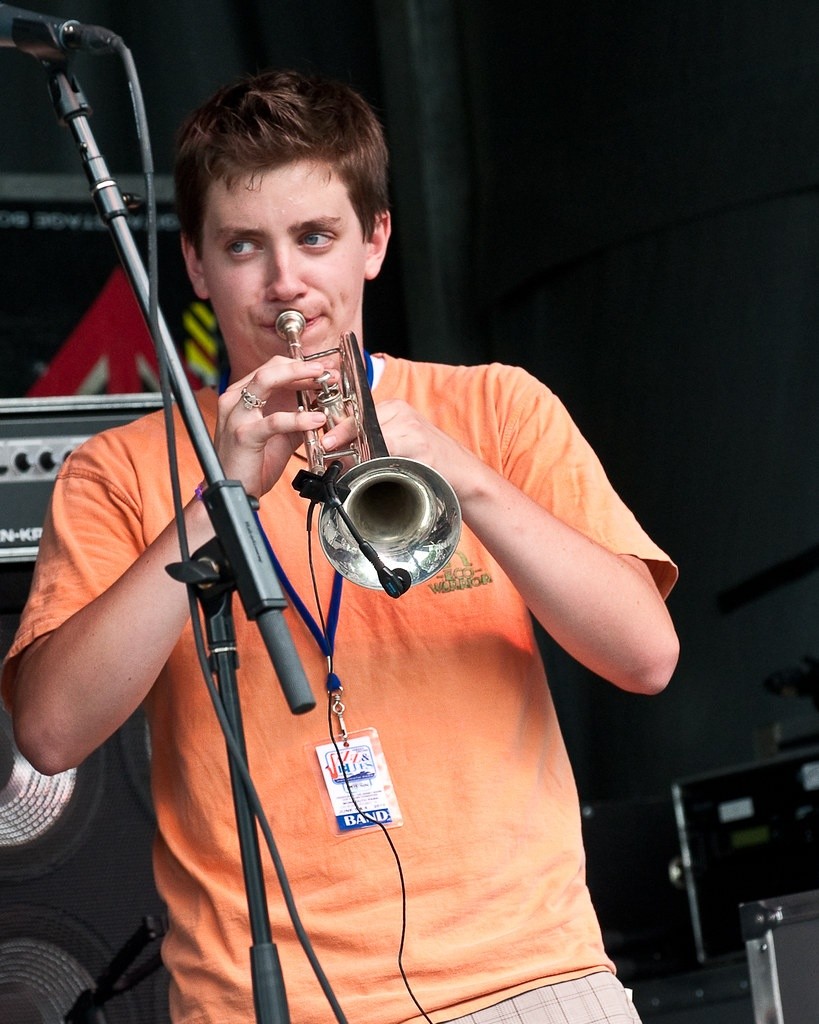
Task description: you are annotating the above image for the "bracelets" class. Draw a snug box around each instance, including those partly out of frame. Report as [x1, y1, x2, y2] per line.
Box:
[194, 484, 203, 498]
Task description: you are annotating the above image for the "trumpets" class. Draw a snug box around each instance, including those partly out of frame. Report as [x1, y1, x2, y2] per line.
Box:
[272, 304, 465, 591]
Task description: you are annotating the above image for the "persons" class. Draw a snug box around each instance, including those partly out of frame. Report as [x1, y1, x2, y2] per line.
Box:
[0, 71, 680, 1024]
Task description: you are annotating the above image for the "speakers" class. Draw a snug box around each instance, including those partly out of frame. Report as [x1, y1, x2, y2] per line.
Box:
[0, 564, 175, 1024]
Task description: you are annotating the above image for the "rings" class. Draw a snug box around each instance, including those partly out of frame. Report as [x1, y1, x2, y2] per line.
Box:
[241, 388, 266, 409]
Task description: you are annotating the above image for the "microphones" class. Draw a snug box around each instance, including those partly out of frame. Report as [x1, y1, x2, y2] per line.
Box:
[0, 4, 123, 57]
[393, 568, 412, 593]
